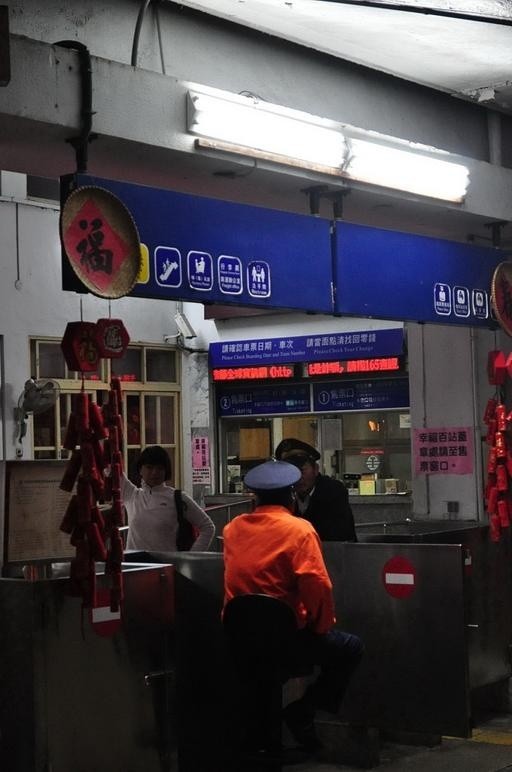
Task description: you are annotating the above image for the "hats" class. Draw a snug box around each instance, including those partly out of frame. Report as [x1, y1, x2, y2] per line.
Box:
[275, 437, 321, 469]
[243, 459, 302, 490]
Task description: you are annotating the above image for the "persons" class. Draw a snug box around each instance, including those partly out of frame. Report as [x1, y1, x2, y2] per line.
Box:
[118, 445, 216, 551]
[220, 459, 366, 749]
[274, 439, 361, 544]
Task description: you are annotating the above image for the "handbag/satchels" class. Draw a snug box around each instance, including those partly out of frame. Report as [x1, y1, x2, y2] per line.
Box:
[174, 489, 196, 551]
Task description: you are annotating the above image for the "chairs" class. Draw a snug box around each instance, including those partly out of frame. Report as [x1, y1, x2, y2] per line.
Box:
[223, 593, 328, 772]
